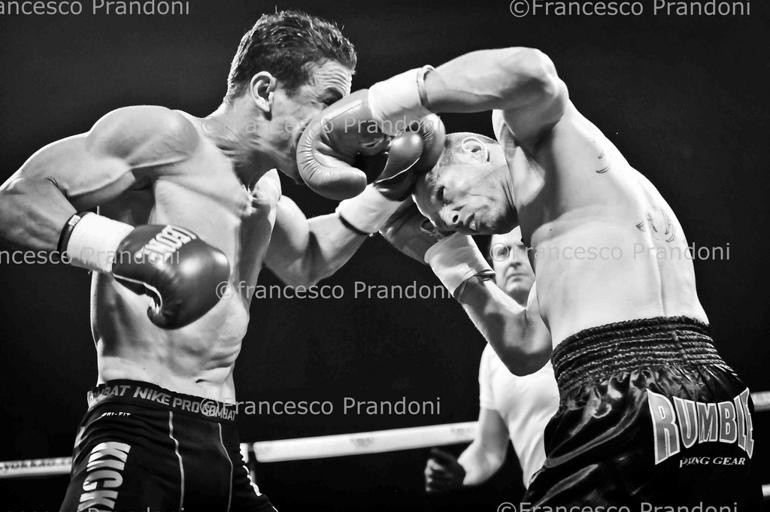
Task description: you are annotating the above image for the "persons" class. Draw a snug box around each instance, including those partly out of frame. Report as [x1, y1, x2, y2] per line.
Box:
[295, 42, 756, 512]
[422, 227, 560, 495]
[0, 10, 446, 511]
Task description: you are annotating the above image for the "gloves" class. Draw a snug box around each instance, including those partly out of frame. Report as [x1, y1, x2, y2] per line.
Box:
[57, 208, 230, 327]
[378, 195, 496, 305]
[339, 112, 446, 237]
[295, 65, 435, 199]
